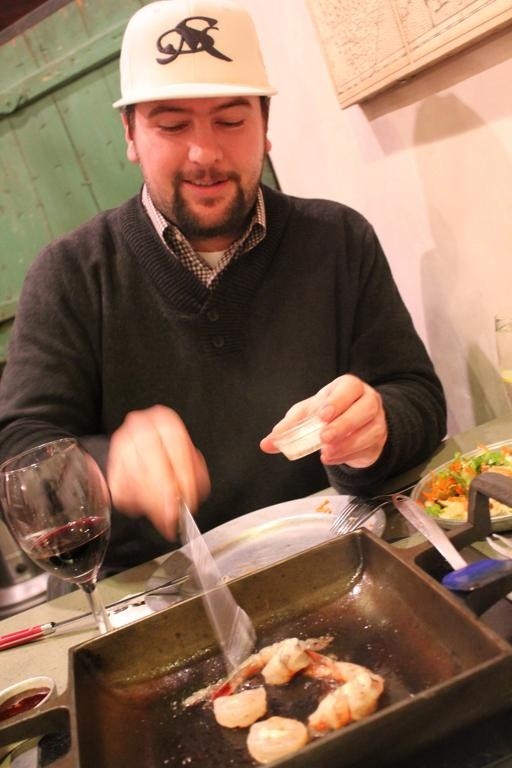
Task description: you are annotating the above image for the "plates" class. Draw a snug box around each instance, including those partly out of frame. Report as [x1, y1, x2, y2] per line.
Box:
[0, 676, 57, 726]
[142, 495, 389, 612]
[412, 437, 512, 533]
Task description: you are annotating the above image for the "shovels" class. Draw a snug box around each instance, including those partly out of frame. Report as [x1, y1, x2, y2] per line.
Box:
[391, 494, 512, 591]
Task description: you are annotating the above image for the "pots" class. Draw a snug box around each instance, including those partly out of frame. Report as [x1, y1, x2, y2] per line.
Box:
[66, 474, 511, 768]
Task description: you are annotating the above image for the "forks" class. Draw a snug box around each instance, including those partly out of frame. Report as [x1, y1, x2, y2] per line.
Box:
[327, 472, 426, 536]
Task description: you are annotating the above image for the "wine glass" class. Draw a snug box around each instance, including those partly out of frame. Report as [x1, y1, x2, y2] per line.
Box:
[0, 437, 114, 635]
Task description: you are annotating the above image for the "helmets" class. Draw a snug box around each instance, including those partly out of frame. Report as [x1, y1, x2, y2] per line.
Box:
[111, 0, 278, 108]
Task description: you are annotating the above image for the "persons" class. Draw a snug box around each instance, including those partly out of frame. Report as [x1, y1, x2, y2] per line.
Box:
[1, 1, 448, 600]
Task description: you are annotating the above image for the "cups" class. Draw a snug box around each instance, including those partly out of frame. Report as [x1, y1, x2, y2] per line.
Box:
[268, 412, 329, 463]
